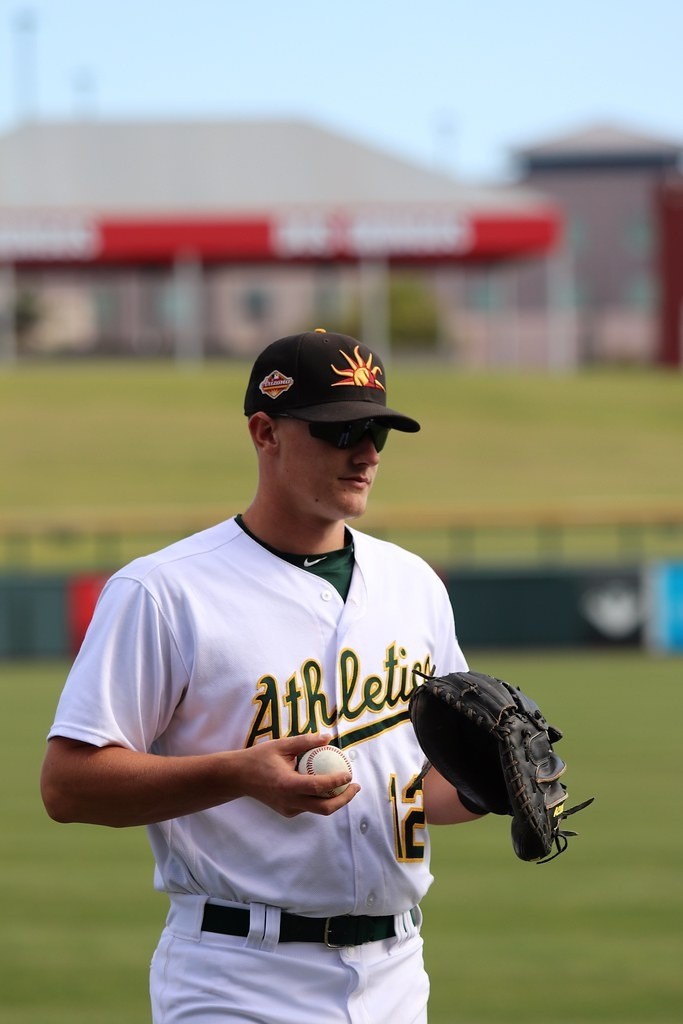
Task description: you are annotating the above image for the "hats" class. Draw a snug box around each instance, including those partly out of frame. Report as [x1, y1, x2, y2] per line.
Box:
[243, 328, 420, 433]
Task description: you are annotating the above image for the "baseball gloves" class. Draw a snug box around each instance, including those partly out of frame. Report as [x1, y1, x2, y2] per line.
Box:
[406, 663, 596, 866]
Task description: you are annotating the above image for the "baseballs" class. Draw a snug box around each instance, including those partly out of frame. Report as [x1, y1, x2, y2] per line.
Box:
[297, 744, 354, 799]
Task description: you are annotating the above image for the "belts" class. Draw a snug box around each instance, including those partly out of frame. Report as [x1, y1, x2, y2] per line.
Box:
[201, 902, 416, 948]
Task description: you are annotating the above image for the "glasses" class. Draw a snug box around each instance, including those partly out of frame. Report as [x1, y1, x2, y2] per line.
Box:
[267, 413, 390, 454]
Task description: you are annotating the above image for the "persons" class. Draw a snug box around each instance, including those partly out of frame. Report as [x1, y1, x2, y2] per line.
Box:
[40, 328, 571, 1024]
